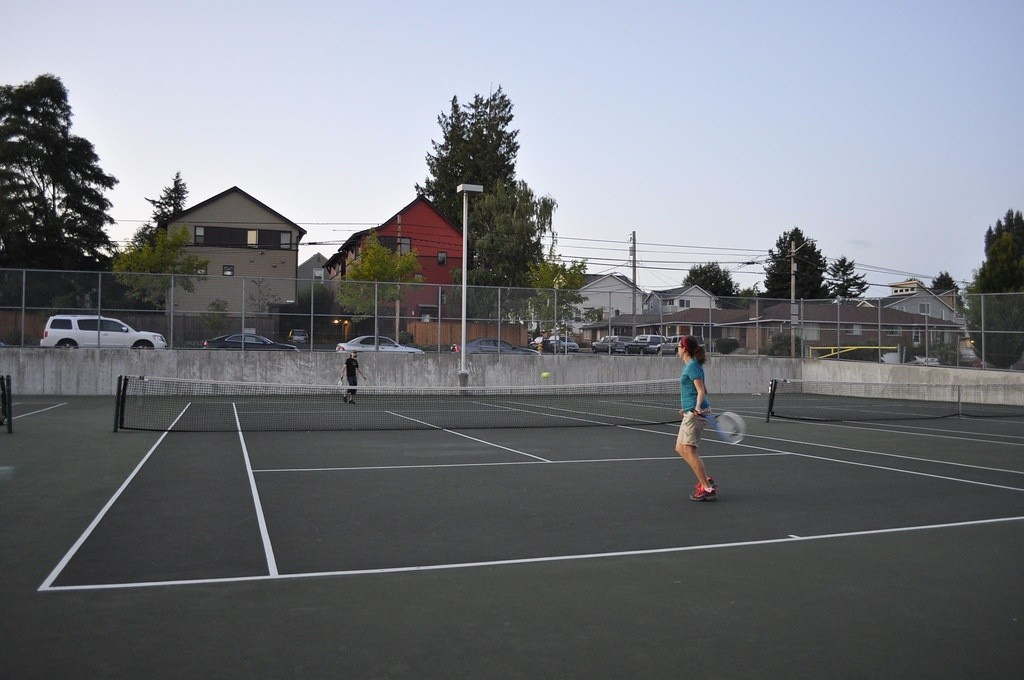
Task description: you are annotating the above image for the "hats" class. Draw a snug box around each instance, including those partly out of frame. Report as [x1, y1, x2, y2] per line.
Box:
[351, 350, 357, 353]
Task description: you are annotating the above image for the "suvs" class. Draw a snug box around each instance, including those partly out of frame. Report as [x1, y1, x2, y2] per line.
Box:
[41, 315, 167, 349]
[625, 335, 666, 354]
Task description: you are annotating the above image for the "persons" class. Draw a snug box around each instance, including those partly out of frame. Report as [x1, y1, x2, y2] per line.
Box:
[340, 349, 367, 404]
[673, 334, 721, 503]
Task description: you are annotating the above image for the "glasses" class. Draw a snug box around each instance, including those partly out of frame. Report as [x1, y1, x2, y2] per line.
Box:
[677, 345, 683, 348]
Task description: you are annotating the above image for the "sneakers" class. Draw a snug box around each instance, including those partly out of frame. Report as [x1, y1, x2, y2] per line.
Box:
[690, 476, 718, 500]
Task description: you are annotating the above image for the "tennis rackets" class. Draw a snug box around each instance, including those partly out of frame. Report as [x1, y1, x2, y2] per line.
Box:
[690, 407, 746, 445]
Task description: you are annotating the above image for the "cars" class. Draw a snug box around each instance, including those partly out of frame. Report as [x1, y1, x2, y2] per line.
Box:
[451, 338, 541, 355]
[336, 336, 425, 354]
[288, 329, 308, 344]
[203, 334, 296, 351]
[529, 337, 549, 347]
[921, 357, 940, 366]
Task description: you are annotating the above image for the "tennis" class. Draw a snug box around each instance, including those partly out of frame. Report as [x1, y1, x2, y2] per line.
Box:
[541, 372, 550, 379]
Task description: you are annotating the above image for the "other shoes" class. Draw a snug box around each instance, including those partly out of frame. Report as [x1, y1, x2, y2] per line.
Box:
[343, 396, 347, 403]
[348, 400, 358, 404]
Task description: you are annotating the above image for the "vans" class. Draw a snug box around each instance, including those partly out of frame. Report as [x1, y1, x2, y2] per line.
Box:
[549, 336, 579, 351]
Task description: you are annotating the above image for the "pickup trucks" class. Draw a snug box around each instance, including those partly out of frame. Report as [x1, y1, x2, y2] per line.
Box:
[592, 336, 633, 353]
[657, 336, 705, 356]
